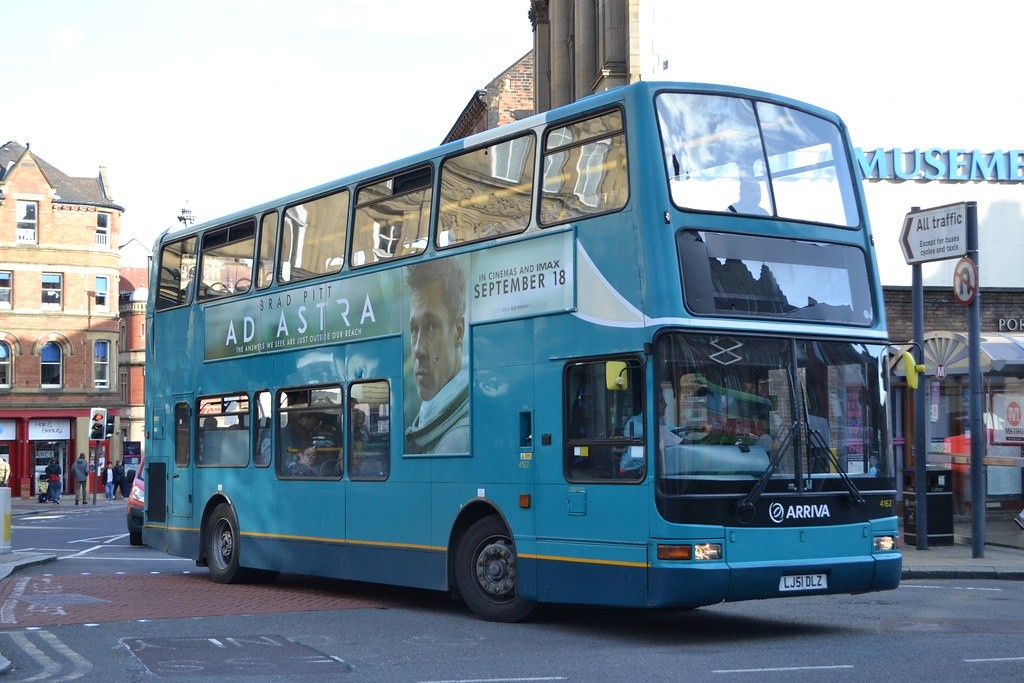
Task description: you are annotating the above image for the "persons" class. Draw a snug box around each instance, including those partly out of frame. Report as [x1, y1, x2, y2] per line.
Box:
[177, 265, 217, 301]
[724, 176, 771, 222]
[619, 385, 713, 476]
[257, 416, 271, 431]
[228, 422, 249, 433]
[0, 454, 13, 487]
[113, 458, 124, 498]
[45, 457, 64, 504]
[199, 417, 221, 431]
[71, 452, 89, 504]
[285, 396, 368, 470]
[402, 254, 471, 457]
[101, 461, 115, 502]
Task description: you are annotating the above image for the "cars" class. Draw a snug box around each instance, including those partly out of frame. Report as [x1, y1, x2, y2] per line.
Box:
[126, 456, 145, 545]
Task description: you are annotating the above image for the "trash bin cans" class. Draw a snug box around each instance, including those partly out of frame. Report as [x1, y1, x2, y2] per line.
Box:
[39, 474, 52, 501]
[900, 466, 955, 547]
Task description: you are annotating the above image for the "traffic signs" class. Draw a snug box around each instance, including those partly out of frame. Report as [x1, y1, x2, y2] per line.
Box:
[899, 202, 968, 265]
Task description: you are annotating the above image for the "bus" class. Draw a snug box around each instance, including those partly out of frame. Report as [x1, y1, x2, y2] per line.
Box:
[139, 80, 924, 622]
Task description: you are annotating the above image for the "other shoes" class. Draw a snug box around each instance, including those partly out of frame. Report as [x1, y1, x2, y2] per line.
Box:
[52, 499, 60, 504]
[83, 501, 88, 504]
[75, 500, 79, 505]
[106, 500, 113, 505]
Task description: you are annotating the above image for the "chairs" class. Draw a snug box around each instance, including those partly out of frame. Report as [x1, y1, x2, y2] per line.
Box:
[318, 458, 341, 477]
[176, 254, 343, 306]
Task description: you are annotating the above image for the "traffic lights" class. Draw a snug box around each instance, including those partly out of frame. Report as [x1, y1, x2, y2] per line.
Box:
[90, 408, 106, 441]
